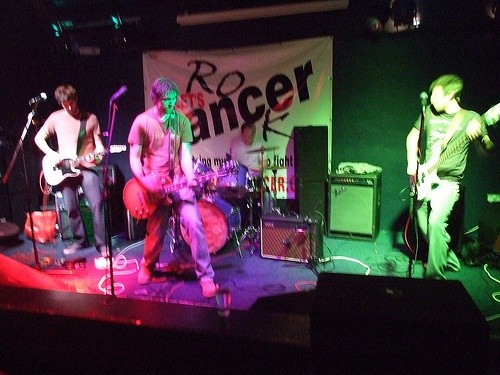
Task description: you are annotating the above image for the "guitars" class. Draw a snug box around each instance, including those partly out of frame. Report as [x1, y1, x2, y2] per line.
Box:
[122, 166, 240, 220]
[411, 103, 500, 200]
[41, 143, 127, 186]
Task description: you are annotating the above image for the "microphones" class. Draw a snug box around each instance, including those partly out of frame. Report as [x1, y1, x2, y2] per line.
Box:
[110, 85, 127, 101]
[419, 91, 429, 113]
[27, 92, 47, 105]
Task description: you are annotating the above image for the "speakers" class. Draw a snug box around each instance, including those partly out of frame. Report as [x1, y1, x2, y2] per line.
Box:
[326, 171, 382, 242]
[259, 214, 323, 264]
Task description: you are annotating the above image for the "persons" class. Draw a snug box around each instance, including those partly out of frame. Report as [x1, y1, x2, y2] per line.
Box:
[230, 122, 268, 176]
[34, 84, 110, 258]
[127, 78, 220, 298]
[406, 74, 495, 279]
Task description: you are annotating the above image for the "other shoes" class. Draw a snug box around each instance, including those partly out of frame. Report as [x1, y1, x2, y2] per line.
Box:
[100, 245, 109, 257]
[138, 267, 152, 284]
[200, 278, 217, 297]
[424, 262, 449, 272]
[64, 242, 88, 254]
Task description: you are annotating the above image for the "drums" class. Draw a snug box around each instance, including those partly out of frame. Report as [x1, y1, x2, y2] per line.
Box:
[216, 162, 249, 199]
[179, 196, 242, 253]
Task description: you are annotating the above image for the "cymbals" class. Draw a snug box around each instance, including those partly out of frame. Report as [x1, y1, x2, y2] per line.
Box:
[247, 146, 278, 154]
[265, 166, 287, 169]
